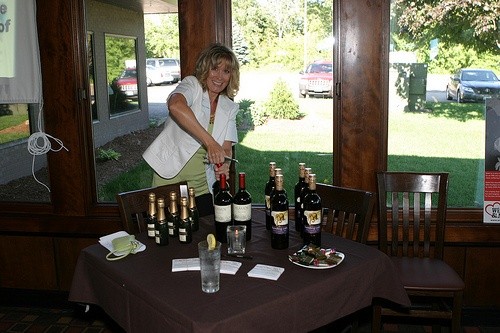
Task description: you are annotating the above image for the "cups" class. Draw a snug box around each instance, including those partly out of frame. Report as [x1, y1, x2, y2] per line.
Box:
[198, 241, 222, 293]
[227, 225, 247, 254]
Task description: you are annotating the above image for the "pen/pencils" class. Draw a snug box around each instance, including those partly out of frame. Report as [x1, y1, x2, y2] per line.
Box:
[226, 254, 253, 260]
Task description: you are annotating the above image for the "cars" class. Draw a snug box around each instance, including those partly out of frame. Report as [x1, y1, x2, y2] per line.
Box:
[117, 68, 138, 101]
[446, 69, 500, 103]
[299, 60, 333, 98]
[146, 65, 174, 86]
[146, 58, 181, 83]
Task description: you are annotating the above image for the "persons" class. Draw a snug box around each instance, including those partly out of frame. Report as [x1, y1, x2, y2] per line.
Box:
[141, 44, 241, 220]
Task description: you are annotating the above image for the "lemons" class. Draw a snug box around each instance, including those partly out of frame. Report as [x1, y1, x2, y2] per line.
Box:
[207, 234, 216, 250]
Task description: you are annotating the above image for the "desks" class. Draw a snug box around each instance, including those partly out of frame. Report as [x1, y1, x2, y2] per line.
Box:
[68, 210, 411, 333]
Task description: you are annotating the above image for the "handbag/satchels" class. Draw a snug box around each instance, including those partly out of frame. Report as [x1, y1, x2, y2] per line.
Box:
[106, 234, 139, 260]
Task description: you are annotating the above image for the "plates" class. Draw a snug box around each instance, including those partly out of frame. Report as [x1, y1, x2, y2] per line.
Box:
[289, 248, 345, 269]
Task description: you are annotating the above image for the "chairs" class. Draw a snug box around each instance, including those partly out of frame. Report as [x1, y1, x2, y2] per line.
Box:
[311, 180, 376, 244]
[115, 182, 191, 235]
[368, 171, 466, 333]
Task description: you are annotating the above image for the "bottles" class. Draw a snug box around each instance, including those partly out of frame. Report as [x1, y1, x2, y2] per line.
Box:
[178, 196, 193, 243]
[212, 163, 230, 197]
[214, 174, 232, 243]
[232, 172, 252, 241]
[264, 161, 322, 249]
[166, 190, 179, 236]
[145, 192, 157, 238]
[187, 187, 199, 233]
[154, 197, 169, 246]
